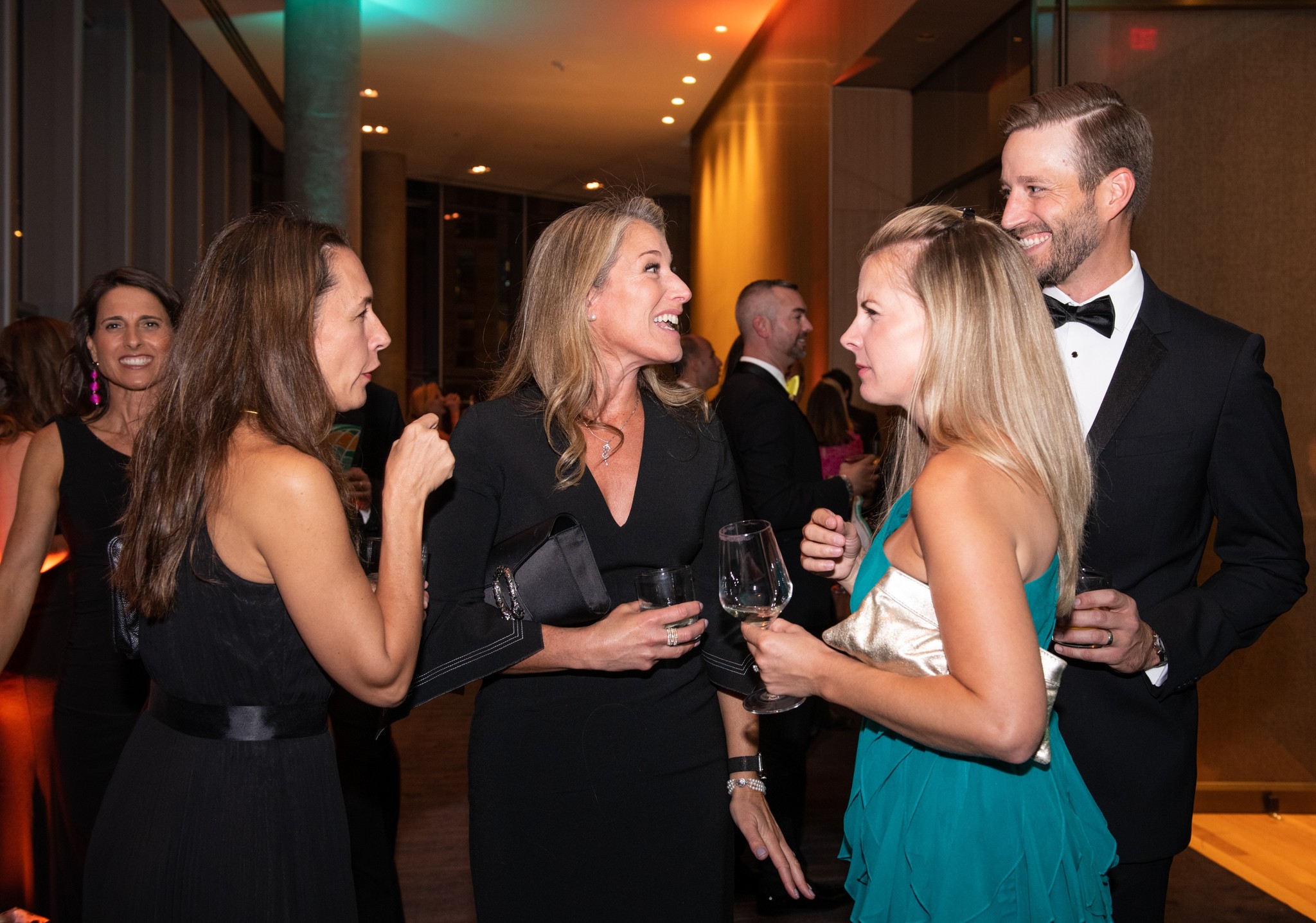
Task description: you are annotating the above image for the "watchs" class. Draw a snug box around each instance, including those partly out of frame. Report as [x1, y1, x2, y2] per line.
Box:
[1152, 629, 1169, 668]
[727, 753, 765, 778]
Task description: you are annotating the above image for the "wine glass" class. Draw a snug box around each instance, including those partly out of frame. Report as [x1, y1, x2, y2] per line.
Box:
[718, 519, 806, 714]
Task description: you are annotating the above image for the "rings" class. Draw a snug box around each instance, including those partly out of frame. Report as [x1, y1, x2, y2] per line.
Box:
[666, 627, 678, 645]
[1102, 628, 1112, 646]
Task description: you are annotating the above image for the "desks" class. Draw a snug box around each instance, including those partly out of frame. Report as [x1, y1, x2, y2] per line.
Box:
[1165, 782, 1314, 922]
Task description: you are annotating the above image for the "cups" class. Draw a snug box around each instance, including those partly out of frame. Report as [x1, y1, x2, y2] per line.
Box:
[635, 564, 701, 646]
[1051, 567, 1113, 648]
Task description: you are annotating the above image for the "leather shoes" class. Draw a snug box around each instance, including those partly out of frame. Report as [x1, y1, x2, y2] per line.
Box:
[755, 878, 852, 915]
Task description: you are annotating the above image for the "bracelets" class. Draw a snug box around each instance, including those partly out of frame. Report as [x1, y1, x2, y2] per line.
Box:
[726, 778, 767, 796]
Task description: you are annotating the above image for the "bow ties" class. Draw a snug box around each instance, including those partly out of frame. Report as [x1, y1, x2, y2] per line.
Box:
[785, 374, 800, 396]
[1041, 292, 1116, 339]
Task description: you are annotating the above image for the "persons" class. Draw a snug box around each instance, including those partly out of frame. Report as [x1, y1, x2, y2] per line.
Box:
[3, 311, 890, 836]
[998, 76, 1311, 922]
[734, 204, 1126, 923]
[1, 262, 193, 922]
[117, 212, 456, 922]
[426, 192, 820, 923]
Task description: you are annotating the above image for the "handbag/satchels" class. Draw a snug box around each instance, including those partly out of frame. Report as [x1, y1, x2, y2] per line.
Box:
[822, 565, 1070, 765]
[483, 512, 613, 626]
[107, 533, 145, 661]
